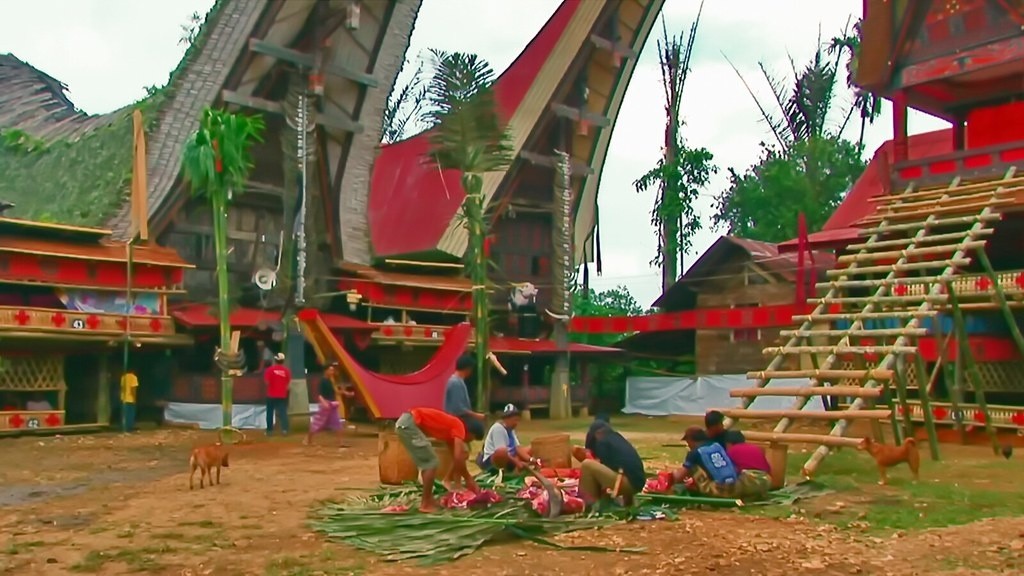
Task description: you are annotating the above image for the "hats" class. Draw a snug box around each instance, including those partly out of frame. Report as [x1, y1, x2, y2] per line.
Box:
[502, 403, 520, 417]
[273, 353, 286, 360]
[588, 419, 607, 440]
[681, 426, 703, 440]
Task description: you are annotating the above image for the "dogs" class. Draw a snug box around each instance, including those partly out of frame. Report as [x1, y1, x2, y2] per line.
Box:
[189, 446, 230, 490]
[855, 436, 920, 486]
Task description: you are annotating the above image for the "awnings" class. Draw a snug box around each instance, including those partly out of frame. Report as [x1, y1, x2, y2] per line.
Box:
[487, 335, 625, 352]
[167, 303, 381, 329]
[777, 228, 872, 255]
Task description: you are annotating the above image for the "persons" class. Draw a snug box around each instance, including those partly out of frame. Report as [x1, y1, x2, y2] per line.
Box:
[644, 410, 772, 498]
[263, 352, 292, 437]
[253, 340, 275, 376]
[577, 414, 646, 503]
[119, 368, 139, 433]
[395, 408, 485, 513]
[481, 403, 541, 475]
[317, 364, 336, 432]
[440, 353, 487, 493]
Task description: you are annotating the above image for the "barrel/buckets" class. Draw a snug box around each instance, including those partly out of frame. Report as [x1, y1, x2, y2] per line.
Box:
[759, 445, 788, 489]
[530, 434, 573, 469]
[377, 432, 419, 486]
